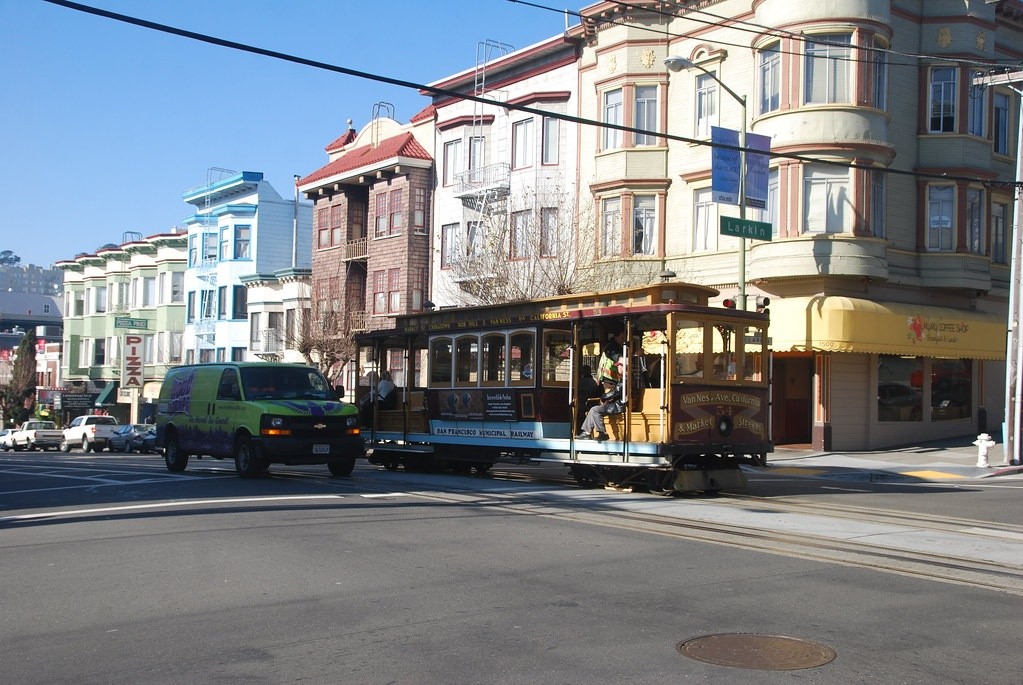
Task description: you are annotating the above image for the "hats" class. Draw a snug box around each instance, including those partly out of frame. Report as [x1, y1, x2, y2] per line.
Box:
[613, 357, 628, 367]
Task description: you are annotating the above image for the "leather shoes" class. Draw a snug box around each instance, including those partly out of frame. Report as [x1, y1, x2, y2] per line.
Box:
[593, 431, 609, 440]
[575, 432, 591, 440]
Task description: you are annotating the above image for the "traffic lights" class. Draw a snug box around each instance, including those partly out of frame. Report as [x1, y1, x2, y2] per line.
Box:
[755, 296, 770, 327]
[722, 297, 737, 311]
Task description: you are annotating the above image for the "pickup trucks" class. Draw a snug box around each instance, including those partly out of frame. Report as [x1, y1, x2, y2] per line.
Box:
[11, 420, 63, 451]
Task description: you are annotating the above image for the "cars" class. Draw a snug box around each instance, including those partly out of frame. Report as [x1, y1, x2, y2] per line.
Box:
[107, 424, 153, 453]
[0, 429, 19, 451]
[144, 429, 166, 457]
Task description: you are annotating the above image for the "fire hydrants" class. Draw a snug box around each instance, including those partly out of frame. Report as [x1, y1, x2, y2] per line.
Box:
[972, 433, 996, 468]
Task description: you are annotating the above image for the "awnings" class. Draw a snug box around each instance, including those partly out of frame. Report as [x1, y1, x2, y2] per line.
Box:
[642, 292, 1007, 361]
[95, 381, 119, 406]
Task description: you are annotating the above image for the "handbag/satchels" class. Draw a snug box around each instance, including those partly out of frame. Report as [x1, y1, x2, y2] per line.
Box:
[384, 386, 398, 403]
[358, 395, 371, 411]
[598, 387, 616, 415]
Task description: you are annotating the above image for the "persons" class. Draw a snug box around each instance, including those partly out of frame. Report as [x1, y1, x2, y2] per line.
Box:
[580, 329, 660, 439]
[372, 371, 395, 400]
[678, 355, 704, 377]
[433, 343, 477, 381]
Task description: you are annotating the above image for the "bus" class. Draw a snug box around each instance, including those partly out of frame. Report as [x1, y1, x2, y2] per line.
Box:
[350, 269, 772, 495]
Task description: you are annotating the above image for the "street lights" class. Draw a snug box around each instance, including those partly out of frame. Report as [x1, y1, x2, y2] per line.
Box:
[662, 54, 749, 310]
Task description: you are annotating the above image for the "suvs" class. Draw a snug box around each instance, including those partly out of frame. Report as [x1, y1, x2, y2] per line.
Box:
[59, 414, 118, 454]
[152, 360, 360, 479]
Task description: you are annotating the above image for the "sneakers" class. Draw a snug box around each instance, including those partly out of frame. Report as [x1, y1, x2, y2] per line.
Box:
[359, 425, 372, 430]
